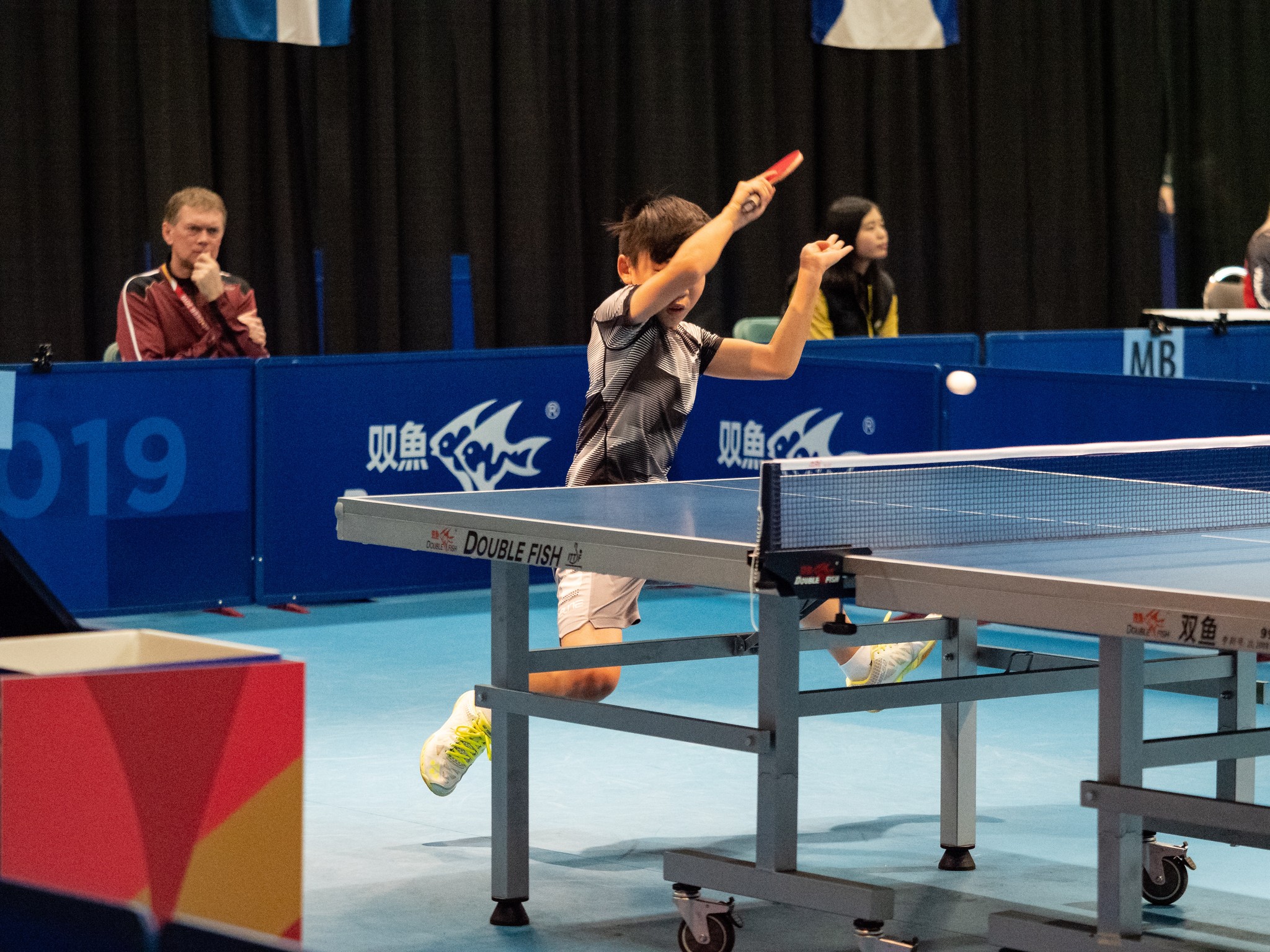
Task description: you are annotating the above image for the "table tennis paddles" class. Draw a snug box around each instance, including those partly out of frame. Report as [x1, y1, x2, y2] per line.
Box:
[740, 150, 804, 214]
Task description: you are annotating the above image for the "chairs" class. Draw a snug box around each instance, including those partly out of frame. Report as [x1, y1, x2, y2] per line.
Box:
[1204, 266, 1248, 308]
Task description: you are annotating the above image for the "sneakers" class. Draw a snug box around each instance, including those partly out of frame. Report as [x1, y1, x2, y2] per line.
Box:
[846, 608, 944, 712]
[420, 689, 498, 796]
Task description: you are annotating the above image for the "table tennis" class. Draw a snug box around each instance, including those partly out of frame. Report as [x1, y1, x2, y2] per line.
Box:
[945, 370, 976, 396]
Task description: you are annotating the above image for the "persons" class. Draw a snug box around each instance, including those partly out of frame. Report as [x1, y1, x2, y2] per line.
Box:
[420, 170, 945, 797]
[116, 188, 269, 361]
[1244, 210, 1270, 311]
[781, 196, 898, 341]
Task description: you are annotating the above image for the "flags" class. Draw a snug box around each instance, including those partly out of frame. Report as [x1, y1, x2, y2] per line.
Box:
[810, 0, 960, 49]
[210, 0, 349, 47]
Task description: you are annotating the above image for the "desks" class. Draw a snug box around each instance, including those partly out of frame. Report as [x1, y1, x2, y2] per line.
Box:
[337, 434, 1270, 952]
